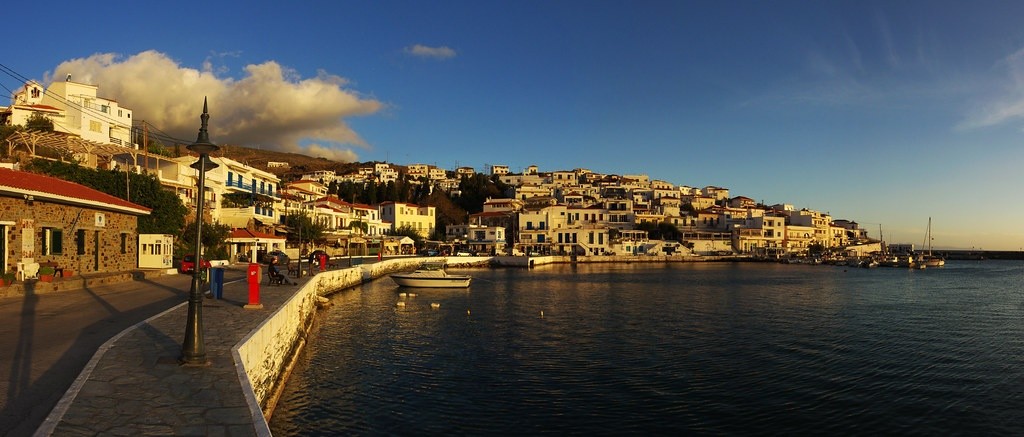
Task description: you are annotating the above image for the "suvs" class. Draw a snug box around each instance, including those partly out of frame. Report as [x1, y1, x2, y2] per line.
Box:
[420, 249, 438, 257]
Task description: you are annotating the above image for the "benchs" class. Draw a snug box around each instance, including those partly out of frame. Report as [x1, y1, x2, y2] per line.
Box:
[328, 261, 338, 269]
[288, 264, 298, 276]
[268, 271, 279, 286]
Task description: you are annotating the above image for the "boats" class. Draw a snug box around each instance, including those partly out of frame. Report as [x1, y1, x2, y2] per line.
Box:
[779, 254, 927, 271]
[389, 263, 475, 289]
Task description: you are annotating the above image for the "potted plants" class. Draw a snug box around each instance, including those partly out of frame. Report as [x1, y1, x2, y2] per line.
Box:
[38, 267, 55, 282]
[0, 272, 16, 286]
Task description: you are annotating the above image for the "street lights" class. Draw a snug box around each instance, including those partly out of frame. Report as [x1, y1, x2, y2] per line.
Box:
[179, 96, 221, 369]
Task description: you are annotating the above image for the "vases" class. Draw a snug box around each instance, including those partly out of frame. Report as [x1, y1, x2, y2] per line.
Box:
[62, 271, 73, 276]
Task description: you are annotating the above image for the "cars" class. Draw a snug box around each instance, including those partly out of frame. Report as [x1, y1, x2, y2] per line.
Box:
[182, 253, 210, 274]
[475, 251, 491, 257]
[530, 251, 542, 257]
[263, 251, 292, 265]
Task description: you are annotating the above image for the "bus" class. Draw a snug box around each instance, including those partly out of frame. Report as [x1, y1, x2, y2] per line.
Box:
[361, 239, 381, 256]
[419, 240, 444, 253]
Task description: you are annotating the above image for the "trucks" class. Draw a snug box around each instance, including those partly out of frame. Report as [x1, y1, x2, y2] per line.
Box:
[495, 249, 509, 256]
[512, 249, 526, 257]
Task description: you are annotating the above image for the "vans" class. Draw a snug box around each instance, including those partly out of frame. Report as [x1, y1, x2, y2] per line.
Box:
[456, 250, 472, 257]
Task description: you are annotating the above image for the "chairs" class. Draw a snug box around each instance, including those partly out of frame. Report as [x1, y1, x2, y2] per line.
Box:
[17, 262, 40, 282]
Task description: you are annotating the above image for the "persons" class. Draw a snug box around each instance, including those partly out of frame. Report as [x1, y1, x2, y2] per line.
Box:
[268, 256, 298, 286]
[318, 252, 330, 268]
[308, 251, 315, 276]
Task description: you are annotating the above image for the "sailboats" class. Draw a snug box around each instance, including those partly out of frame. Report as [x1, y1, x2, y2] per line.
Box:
[923, 216, 945, 266]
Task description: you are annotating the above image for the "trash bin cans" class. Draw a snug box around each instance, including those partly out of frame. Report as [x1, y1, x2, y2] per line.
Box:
[209, 268, 225, 299]
